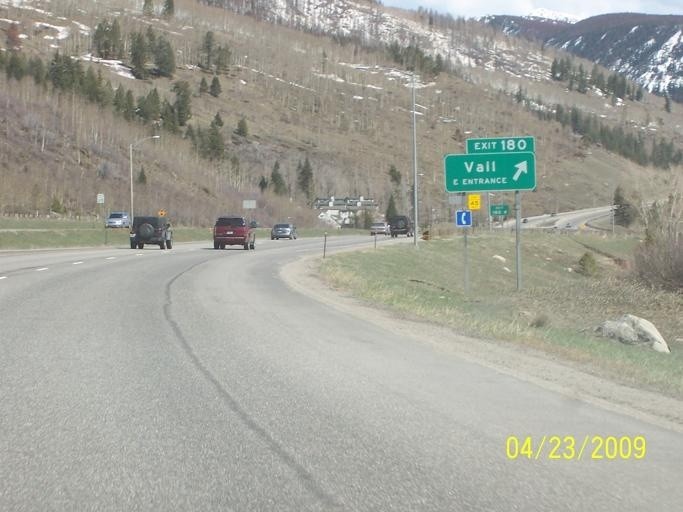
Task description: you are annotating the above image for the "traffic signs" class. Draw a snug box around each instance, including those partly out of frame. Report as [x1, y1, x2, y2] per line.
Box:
[444, 136, 537, 294]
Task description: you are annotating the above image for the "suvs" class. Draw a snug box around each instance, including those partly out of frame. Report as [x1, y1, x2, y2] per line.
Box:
[391, 214, 415, 238]
[128, 216, 175, 250]
[105, 211, 129, 228]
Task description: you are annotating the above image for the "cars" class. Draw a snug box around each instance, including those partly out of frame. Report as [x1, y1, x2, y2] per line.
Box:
[213, 216, 258, 250]
[270, 222, 298, 240]
[370, 221, 390, 236]
